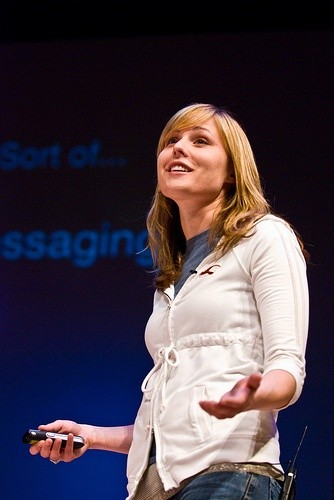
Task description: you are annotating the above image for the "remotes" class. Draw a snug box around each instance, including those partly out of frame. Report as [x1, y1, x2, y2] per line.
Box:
[23, 428, 85, 451]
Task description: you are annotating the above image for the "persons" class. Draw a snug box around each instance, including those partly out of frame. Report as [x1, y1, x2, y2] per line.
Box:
[28, 101, 310, 499]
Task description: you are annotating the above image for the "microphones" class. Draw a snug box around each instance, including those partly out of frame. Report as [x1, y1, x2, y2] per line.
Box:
[189, 269, 197, 273]
